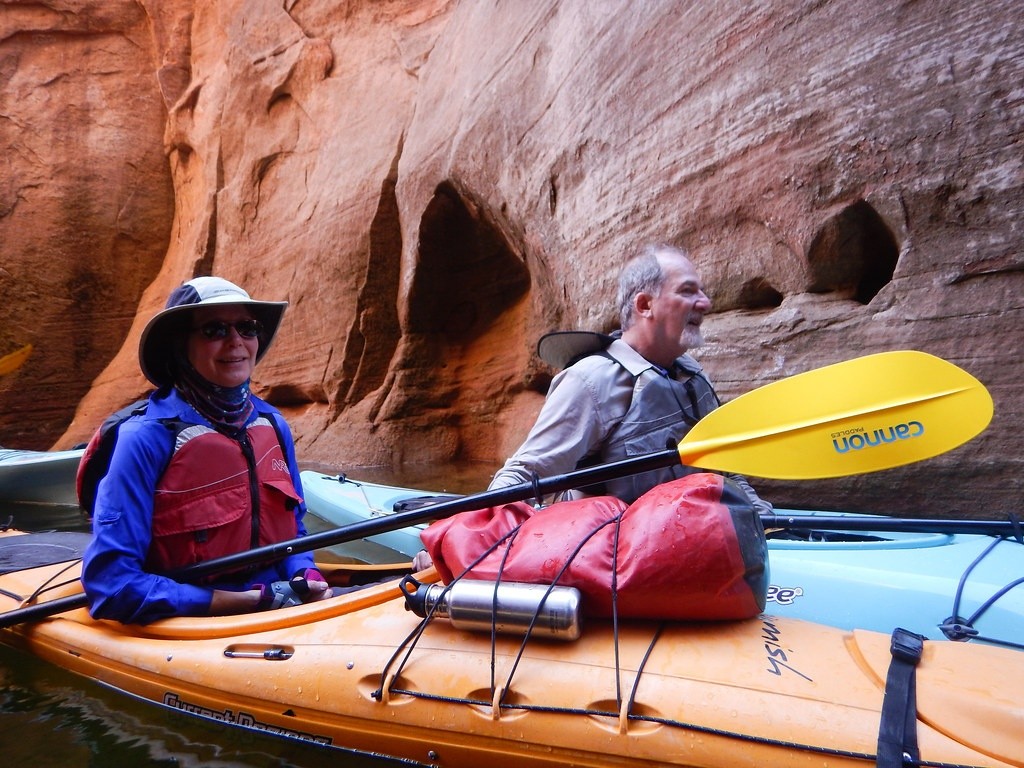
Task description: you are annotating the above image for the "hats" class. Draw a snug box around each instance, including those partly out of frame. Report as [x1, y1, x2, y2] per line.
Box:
[138, 276, 288, 388]
[537, 329, 623, 370]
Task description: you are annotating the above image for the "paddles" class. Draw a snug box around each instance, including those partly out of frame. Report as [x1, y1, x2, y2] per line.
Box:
[0, 348, 996, 630]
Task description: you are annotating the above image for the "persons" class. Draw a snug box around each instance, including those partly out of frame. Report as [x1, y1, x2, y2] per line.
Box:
[412, 247, 798, 573]
[76, 277, 381, 625]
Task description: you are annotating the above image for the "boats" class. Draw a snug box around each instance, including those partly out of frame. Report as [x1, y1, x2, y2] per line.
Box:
[297, 467, 1023, 650]
[0, 526, 1023, 768]
[0, 442, 88, 521]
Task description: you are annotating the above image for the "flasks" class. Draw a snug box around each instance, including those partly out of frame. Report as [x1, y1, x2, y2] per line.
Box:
[399, 574, 581, 642]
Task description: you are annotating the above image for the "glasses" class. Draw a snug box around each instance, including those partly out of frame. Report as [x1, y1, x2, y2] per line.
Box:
[186, 317, 264, 340]
